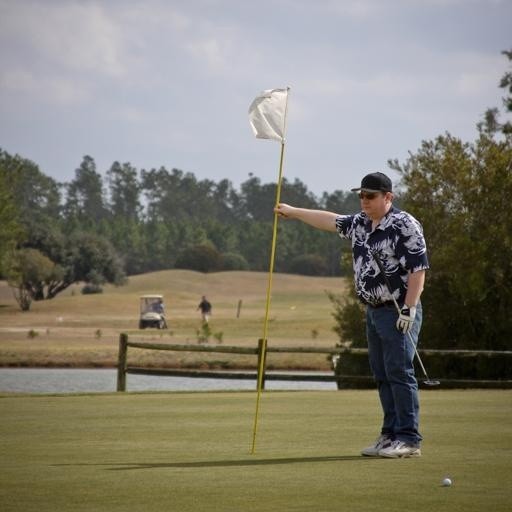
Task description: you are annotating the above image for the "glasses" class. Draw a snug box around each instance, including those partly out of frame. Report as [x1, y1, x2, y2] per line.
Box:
[359, 194, 378, 199]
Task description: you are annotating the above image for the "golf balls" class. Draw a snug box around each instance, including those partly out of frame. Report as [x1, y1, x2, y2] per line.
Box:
[442, 478, 452, 488]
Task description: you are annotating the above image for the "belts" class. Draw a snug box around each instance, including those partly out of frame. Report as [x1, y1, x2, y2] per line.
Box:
[368, 300, 402, 308]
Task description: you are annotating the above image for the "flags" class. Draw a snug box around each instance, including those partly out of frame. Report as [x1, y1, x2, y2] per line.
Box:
[246, 87, 288, 144]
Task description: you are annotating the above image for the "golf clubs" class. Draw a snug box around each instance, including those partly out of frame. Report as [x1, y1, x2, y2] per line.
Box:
[372, 253, 440, 386]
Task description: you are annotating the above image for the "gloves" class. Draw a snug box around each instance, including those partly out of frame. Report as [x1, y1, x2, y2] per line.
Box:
[396, 304, 417, 335]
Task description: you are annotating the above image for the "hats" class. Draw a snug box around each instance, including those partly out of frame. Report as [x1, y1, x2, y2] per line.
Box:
[351, 172, 392, 193]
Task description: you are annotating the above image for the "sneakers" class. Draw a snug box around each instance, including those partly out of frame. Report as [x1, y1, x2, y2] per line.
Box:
[378, 439, 421, 458]
[362, 434, 395, 456]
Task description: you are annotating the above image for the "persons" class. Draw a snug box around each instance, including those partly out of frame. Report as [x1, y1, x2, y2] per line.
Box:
[273, 171, 432, 458]
[197, 296, 212, 323]
[152, 300, 165, 314]
